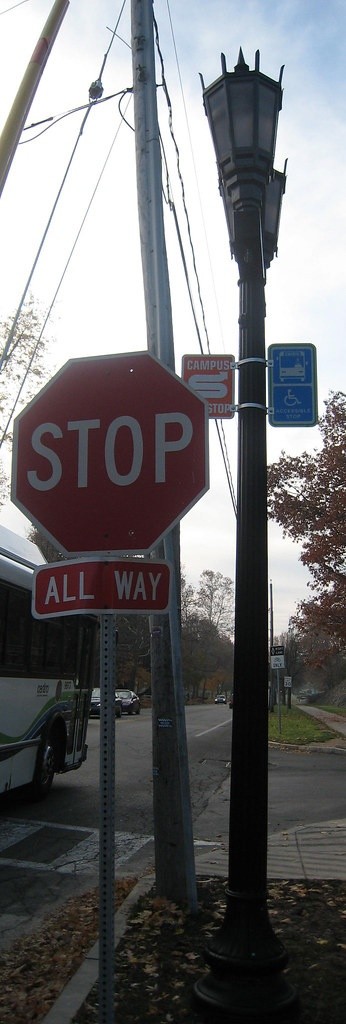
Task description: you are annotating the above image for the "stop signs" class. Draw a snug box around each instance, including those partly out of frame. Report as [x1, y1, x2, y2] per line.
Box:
[8, 351, 210, 556]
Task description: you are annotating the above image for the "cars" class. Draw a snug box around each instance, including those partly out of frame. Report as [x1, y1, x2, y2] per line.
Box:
[229, 690, 233, 709]
[215, 695, 227, 704]
[89, 687, 123, 717]
[115, 689, 141, 715]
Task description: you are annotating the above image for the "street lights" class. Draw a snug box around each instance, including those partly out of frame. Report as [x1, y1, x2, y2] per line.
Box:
[199, 46, 288, 984]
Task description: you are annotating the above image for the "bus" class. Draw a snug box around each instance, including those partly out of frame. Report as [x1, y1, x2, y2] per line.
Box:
[0, 525, 99, 794]
